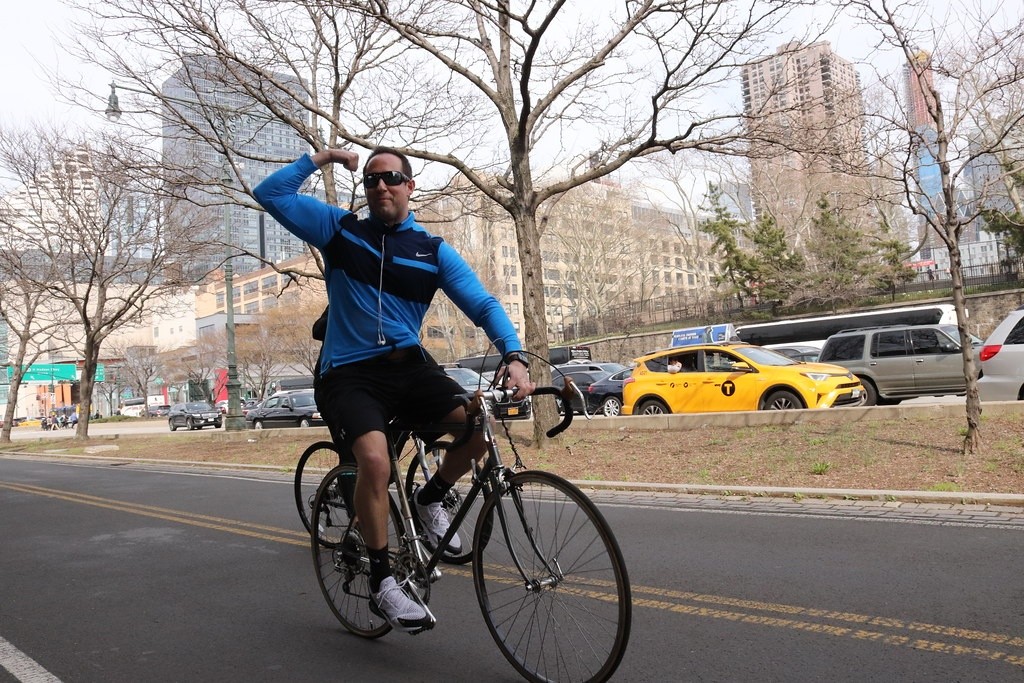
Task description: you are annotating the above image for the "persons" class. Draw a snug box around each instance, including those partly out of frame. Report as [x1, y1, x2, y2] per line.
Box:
[254, 146, 534, 631]
[41, 408, 122, 431]
[667, 355, 688, 372]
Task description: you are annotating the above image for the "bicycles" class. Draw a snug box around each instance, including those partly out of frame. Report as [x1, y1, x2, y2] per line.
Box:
[311, 338, 632, 683]
[294, 432, 493, 565]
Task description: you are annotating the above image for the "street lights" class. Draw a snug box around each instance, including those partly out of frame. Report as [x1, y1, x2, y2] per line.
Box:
[104, 81, 326, 431]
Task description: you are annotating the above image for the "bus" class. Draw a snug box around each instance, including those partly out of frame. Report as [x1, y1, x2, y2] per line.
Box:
[454, 345, 591, 374]
[120, 395, 165, 418]
[735, 304, 957, 347]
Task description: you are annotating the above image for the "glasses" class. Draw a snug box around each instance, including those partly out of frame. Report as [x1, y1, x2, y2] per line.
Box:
[364, 171, 411, 188]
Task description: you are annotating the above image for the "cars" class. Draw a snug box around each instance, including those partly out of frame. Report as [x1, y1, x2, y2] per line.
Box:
[169, 402, 223, 431]
[216, 388, 325, 429]
[439, 340, 868, 423]
[140, 404, 171, 417]
[976, 305, 1024, 401]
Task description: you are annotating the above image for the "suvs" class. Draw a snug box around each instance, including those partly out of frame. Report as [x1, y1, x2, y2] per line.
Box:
[817, 325, 985, 405]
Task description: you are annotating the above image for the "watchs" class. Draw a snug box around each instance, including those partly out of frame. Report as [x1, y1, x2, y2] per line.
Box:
[506, 352, 530, 369]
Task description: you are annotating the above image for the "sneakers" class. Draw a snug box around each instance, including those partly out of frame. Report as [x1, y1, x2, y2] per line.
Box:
[408, 482, 462, 556]
[367, 575, 430, 633]
